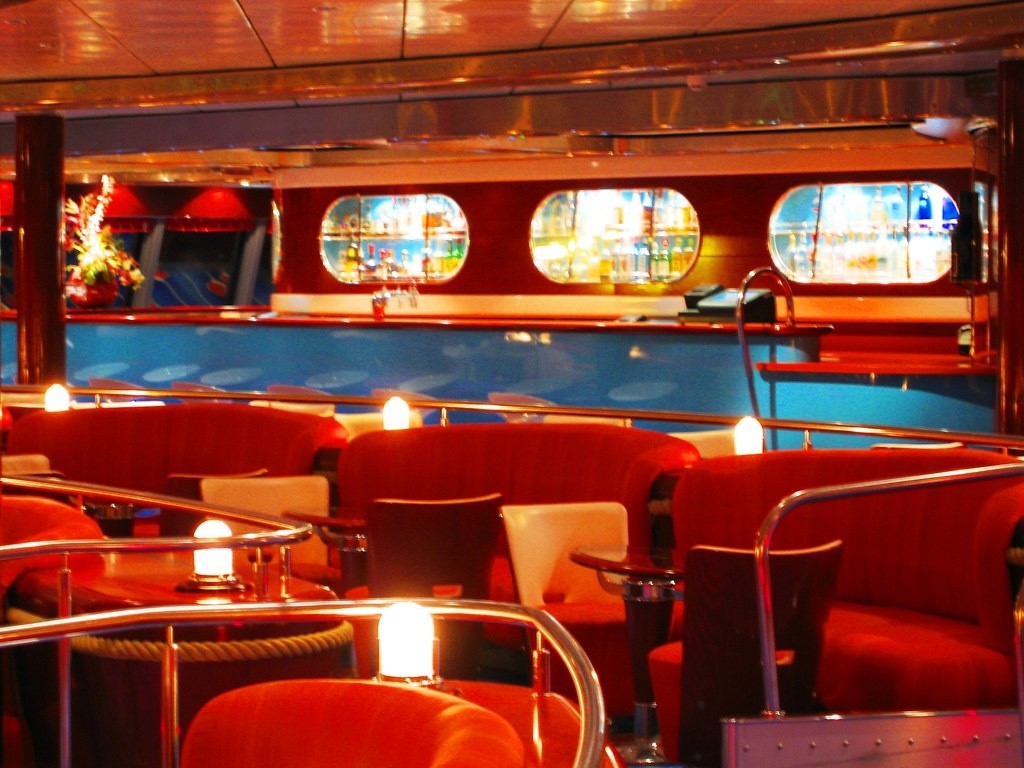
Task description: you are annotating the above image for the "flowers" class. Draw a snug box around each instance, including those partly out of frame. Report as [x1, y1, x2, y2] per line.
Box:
[63, 173, 145, 292]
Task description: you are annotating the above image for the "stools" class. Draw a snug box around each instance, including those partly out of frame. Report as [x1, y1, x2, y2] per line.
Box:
[372, 387, 436, 400]
[488, 391, 557, 423]
[170, 380, 234, 404]
[268, 384, 335, 396]
[88, 378, 150, 403]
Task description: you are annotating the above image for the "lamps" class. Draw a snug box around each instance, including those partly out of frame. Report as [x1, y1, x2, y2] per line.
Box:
[0, 181, 14, 231]
[383, 396, 410, 429]
[372, 601, 463, 697]
[165, 187, 257, 232]
[734, 415, 764, 456]
[81, 184, 153, 233]
[176, 518, 247, 592]
[45, 383, 70, 413]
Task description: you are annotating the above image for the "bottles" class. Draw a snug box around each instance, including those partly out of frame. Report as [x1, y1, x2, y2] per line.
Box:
[324, 196, 464, 280]
[549, 189, 698, 280]
[951, 191, 987, 281]
[786, 185, 959, 281]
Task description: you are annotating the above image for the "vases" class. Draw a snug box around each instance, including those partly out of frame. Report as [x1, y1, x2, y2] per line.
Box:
[67, 267, 120, 309]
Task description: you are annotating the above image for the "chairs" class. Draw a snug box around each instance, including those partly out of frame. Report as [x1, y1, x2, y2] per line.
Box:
[361, 494, 506, 681]
[677, 533, 848, 768]
[159, 469, 270, 536]
[177, 680, 526, 768]
[0, 495, 109, 616]
[201, 473, 332, 567]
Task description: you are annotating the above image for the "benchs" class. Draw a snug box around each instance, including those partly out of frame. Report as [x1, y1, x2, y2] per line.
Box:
[3, 399, 351, 588]
[335, 424, 705, 720]
[645, 447, 1024, 768]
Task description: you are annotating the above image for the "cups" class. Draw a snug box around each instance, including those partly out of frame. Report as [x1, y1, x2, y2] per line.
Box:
[372, 292, 389, 317]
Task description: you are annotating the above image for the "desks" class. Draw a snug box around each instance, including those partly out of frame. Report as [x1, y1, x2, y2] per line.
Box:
[76, 577, 348, 629]
[568, 539, 685, 768]
